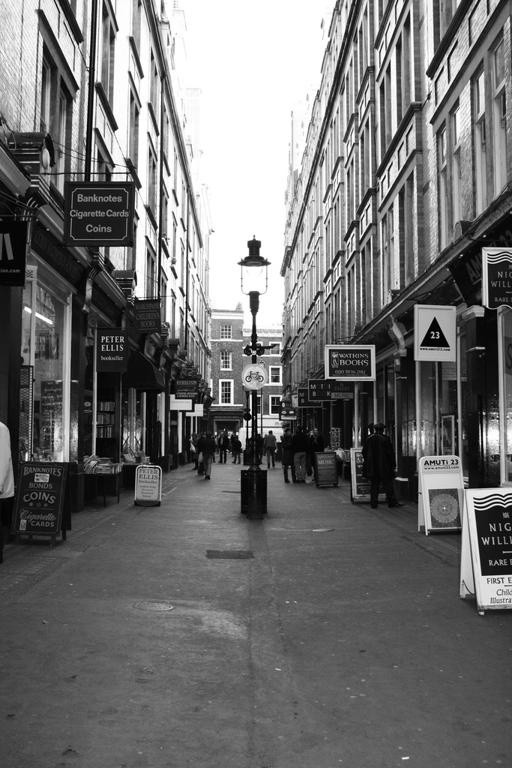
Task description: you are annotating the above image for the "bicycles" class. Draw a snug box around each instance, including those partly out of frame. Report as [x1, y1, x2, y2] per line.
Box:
[244, 369, 264, 384]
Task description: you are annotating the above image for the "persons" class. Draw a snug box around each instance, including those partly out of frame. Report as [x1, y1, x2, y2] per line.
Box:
[1, 422, 16, 565]
[185, 426, 322, 483]
[364, 423, 404, 507]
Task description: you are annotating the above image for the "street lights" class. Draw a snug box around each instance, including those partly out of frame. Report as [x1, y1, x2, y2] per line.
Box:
[238, 235, 270, 521]
[244, 332, 264, 470]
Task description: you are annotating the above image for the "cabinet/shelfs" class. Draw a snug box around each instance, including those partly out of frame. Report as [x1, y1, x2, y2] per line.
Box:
[39, 377, 79, 465]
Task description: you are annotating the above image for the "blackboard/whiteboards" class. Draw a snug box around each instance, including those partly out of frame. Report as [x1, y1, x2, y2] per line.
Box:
[350, 448, 387, 502]
[9, 461, 68, 537]
[314, 452, 338, 484]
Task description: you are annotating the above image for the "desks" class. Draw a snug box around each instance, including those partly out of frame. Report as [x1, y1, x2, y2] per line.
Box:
[78, 470, 124, 509]
[123, 460, 155, 493]
